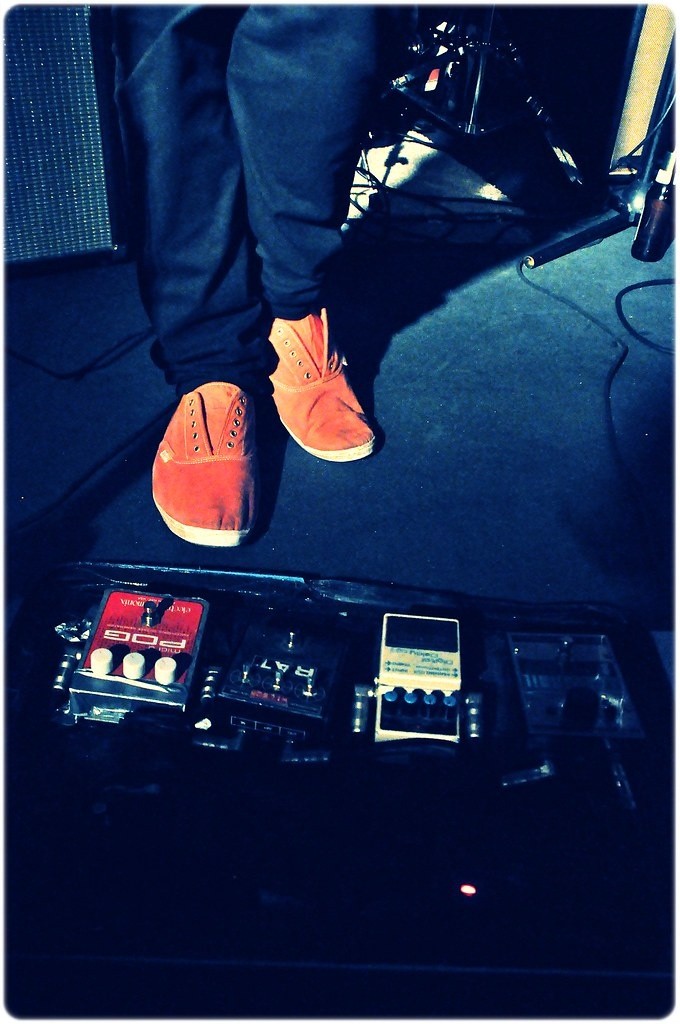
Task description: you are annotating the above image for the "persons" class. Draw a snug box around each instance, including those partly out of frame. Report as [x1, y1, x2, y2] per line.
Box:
[112, 6, 383, 553]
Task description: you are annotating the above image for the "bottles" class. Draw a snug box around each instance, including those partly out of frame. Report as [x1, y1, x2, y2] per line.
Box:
[631, 169, 675, 262]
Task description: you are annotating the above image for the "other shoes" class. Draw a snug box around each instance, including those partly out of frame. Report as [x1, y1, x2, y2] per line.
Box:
[260, 308, 375, 465]
[151, 382, 258, 549]
